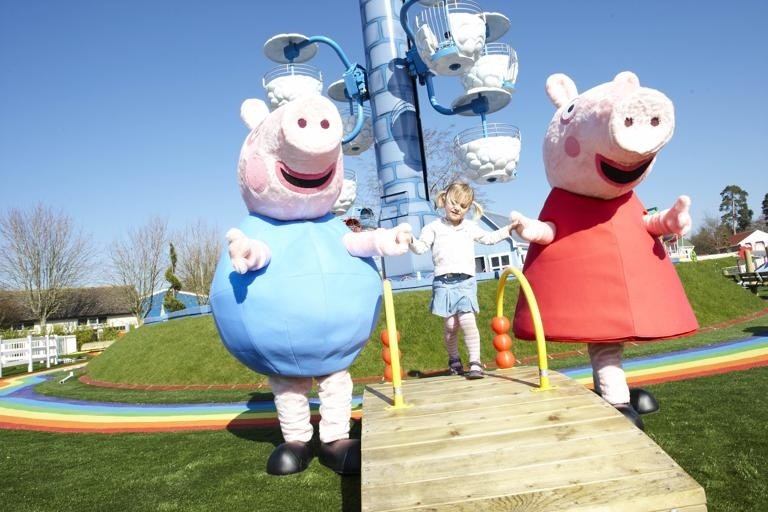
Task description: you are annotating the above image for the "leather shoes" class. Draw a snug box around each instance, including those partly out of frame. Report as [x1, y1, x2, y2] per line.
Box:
[447, 357, 466, 376]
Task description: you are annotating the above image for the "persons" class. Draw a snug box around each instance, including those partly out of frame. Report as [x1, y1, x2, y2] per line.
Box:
[402, 179, 522, 380]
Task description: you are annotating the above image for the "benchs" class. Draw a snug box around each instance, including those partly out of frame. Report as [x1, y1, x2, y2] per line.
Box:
[739, 271, 768, 295]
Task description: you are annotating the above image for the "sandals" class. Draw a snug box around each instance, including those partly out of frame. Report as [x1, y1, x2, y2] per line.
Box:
[469, 363, 487, 378]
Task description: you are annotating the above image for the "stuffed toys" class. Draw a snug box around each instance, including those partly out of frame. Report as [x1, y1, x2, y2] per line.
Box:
[507, 68, 694, 434]
[210, 95, 409, 476]
